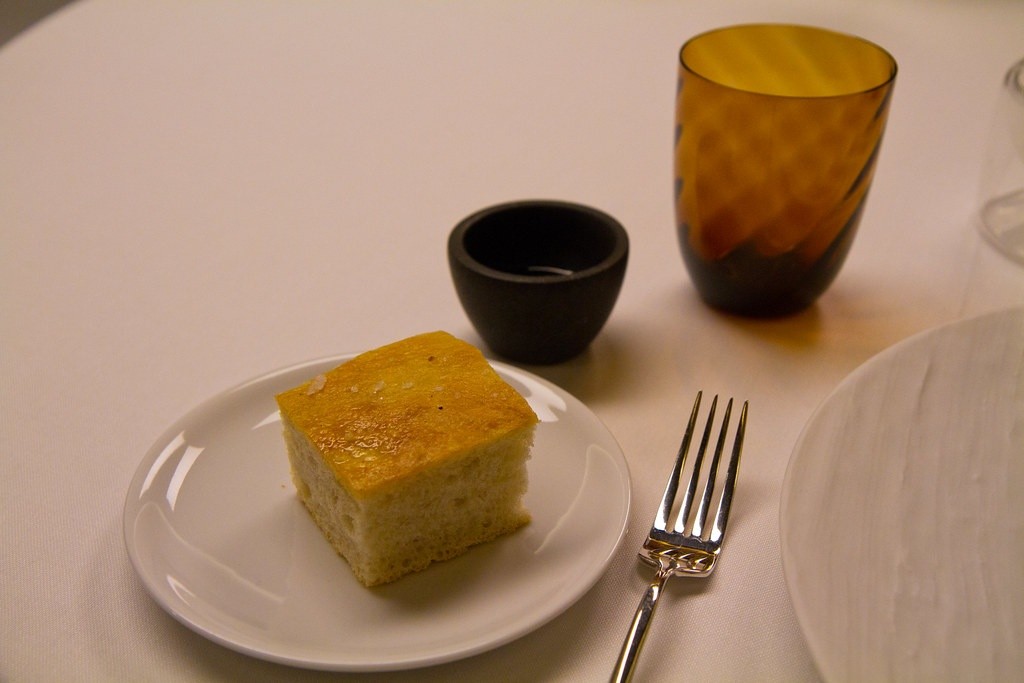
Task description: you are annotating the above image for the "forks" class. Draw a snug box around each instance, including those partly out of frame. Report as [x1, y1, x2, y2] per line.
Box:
[607, 390, 749, 683]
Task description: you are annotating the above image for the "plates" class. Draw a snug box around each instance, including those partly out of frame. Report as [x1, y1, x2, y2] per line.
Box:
[125, 350, 633, 675]
[778, 305, 1024, 683]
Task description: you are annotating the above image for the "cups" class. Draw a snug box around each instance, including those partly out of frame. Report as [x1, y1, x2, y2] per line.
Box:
[446, 200, 630, 367]
[672, 23, 899, 319]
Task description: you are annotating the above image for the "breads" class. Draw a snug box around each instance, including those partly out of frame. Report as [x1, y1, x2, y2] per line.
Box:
[272, 329, 540, 587]
